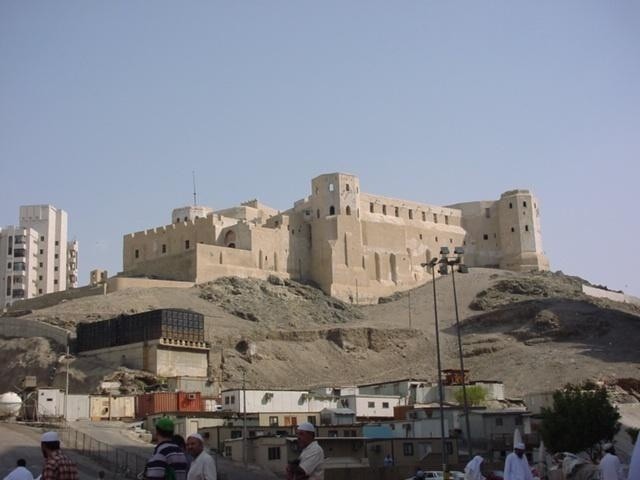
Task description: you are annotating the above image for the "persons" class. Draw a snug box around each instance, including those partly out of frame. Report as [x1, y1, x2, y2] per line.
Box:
[1, 459, 35, 480]
[464, 456, 490, 480]
[284, 423, 326, 479]
[595, 443, 625, 480]
[501, 444, 533, 480]
[139, 417, 217, 479]
[38, 431, 79, 480]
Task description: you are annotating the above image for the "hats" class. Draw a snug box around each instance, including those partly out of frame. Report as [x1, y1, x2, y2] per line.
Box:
[297, 423, 315, 432]
[514, 443, 525, 449]
[603, 443, 613, 451]
[41, 432, 61, 442]
[186, 433, 204, 444]
[157, 418, 175, 432]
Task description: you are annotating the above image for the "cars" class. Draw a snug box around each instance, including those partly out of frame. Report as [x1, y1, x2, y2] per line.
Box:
[407, 471, 464, 480]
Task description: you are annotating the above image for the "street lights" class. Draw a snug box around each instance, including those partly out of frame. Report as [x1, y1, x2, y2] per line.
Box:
[421, 245, 473, 470]
